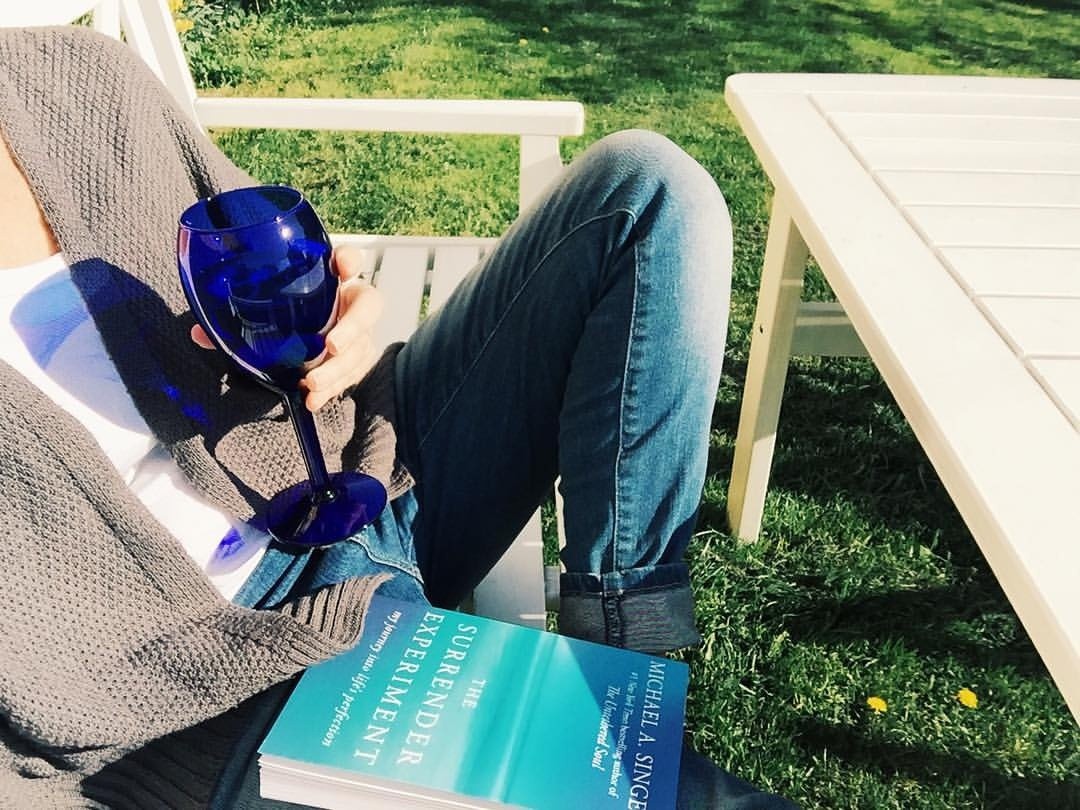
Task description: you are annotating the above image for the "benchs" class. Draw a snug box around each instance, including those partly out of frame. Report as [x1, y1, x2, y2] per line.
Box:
[0, 0, 582, 653]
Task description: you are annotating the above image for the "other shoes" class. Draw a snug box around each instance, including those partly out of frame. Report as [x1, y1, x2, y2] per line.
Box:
[676, 735, 796, 809]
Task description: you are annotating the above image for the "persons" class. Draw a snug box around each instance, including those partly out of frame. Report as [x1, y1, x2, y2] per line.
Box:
[0, 25, 734, 810]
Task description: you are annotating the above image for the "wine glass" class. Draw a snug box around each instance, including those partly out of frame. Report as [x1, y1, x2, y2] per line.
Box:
[178, 186, 387, 550]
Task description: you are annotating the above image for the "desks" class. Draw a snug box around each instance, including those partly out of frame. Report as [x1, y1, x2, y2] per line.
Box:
[724, 73, 1078, 727]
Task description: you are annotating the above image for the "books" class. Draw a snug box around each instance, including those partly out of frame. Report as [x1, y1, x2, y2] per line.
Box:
[256, 594, 688, 810]
